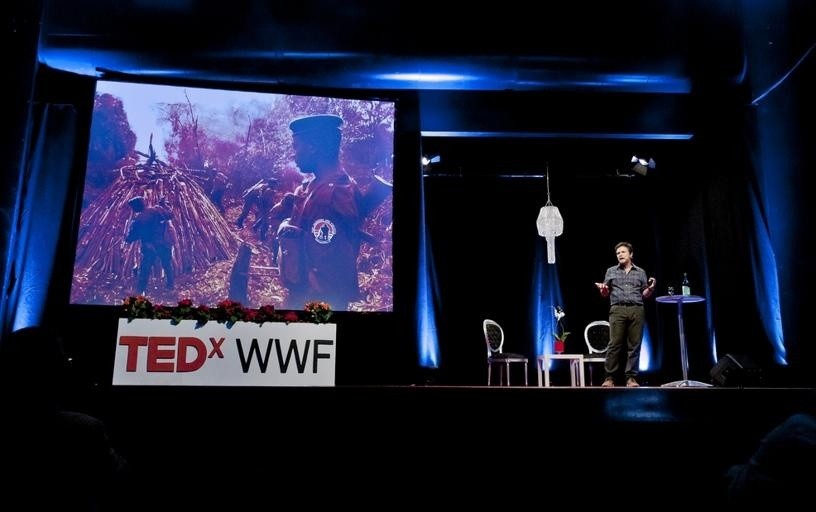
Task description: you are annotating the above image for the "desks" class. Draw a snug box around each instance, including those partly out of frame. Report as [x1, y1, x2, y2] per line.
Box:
[656, 294, 713, 388]
[537, 354, 585, 388]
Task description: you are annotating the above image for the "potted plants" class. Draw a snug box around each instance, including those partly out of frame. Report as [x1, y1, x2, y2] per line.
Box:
[554, 340, 565, 354]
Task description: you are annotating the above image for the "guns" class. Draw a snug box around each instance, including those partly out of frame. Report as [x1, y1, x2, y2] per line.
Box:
[241, 179, 264, 200]
[253, 211, 270, 233]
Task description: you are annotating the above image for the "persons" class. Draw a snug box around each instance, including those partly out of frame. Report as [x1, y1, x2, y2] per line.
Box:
[127, 194, 175, 292]
[253, 180, 311, 231]
[209, 167, 229, 214]
[235, 177, 277, 227]
[0, 325, 128, 512]
[274, 112, 361, 311]
[595, 241, 657, 388]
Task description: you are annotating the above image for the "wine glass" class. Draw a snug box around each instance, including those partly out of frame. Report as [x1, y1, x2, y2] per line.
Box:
[668, 287, 674, 300]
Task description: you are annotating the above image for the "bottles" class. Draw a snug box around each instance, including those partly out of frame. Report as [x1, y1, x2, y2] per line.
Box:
[682, 272, 691, 297]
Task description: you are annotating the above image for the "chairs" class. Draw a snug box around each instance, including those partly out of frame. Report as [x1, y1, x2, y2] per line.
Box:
[575, 321, 611, 387]
[483, 319, 529, 386]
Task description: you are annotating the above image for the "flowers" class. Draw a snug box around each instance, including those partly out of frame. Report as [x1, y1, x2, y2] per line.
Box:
[552, 305, 571, 341]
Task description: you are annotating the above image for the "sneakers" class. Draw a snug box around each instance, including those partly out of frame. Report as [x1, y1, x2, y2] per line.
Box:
[626, 377, 639, 387]
[601, 376, 614, 387]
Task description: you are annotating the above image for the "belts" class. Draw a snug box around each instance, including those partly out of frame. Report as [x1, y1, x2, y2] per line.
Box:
[617, 302, 635, 306]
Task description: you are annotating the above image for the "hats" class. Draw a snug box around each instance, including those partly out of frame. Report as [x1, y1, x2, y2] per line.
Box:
[268, 177, 279, 182]
[289, 115, 344, 136]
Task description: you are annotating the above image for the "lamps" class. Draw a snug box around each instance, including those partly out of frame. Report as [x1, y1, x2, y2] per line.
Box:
[536, 168, 564, 264]
